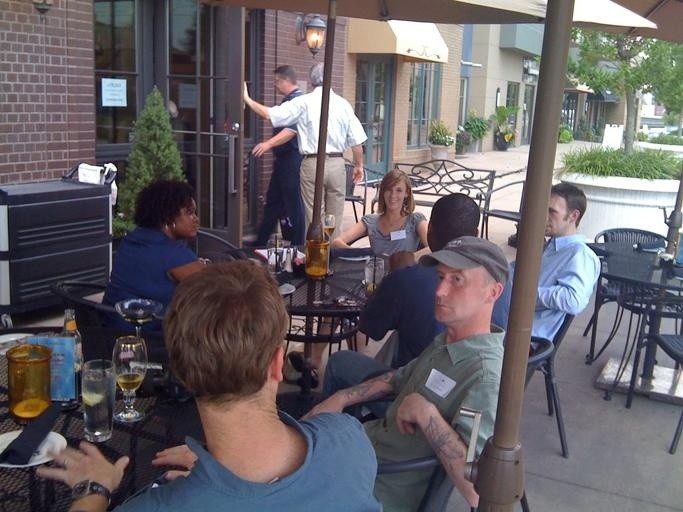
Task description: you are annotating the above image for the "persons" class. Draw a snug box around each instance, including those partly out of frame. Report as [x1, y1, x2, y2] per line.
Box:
[288, 169, 432, 388]
[320, 193, 514, 420]
[35, 258, 384, 512]
[81, 180, 209, 363]
[243, 61, 368, 246]
[298, 235, 506, 512]
[242, 65, 305, 251]
[509, 181, 602, 357]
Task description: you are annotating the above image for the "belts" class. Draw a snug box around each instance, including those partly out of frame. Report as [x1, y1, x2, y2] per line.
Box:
[304, 152, 343, 158]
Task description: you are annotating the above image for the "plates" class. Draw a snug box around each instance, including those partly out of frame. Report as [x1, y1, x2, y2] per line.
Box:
[278, 282, 296, 295]
[0, 426, 70, 469]
[337, 256, 372, 262]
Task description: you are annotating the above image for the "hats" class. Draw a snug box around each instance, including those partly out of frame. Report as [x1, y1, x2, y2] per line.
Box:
[418, 235, 510, 285]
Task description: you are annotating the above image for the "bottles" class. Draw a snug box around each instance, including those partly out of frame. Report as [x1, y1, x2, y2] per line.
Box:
[675, 233, 683, 268]
[284, 249, 293, 273]
[59, 309, 82, 407]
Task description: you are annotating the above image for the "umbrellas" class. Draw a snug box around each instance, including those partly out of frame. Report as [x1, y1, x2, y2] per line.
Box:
[570, 1, 683, 380]
[201, 0, 658, 400]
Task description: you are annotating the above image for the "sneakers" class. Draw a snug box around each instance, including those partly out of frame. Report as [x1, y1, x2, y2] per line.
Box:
[243, 238, 268, 249]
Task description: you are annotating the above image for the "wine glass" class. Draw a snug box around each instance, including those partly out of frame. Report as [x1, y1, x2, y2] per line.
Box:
[111, 337, 148, 424]
[113, 300, 163, 338]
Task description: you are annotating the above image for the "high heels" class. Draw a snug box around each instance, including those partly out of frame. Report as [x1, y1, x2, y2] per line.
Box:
[288, 351, 320, 389]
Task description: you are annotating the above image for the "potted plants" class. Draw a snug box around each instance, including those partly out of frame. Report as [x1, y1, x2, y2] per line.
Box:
[463, 108, 487, 152]
[489, 105, 520, 150]
[428, 119, 455, 169]
[456, 125, 470, 154]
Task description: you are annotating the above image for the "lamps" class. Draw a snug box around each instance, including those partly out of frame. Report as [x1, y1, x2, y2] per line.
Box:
[294, 13, 327, 59]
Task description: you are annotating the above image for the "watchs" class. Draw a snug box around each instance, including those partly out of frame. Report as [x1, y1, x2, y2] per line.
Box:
[71, 479, 112, 507]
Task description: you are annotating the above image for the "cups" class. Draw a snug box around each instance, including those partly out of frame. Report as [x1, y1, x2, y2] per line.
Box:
[267, 238, 296, 273]
[5, 342, 51, 419]
[363, 257, 384, 295]
[305, 239, 331, 278]
[80, 360, 119, 445]
[318, 212, 336, 243]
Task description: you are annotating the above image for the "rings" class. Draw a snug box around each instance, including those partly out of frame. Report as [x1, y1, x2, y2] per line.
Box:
[64, 456, 70, 467]
